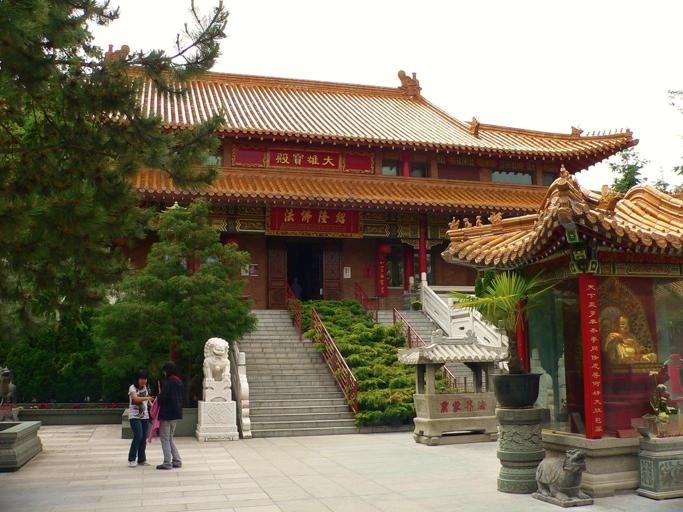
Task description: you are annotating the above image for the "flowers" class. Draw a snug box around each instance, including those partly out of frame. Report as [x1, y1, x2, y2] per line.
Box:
[643, 370, 679, 425]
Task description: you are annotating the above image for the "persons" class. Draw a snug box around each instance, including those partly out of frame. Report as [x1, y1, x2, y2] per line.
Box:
[149, 360, 186, 471]
[605, 314, 657, 364]
[127, 369, 157, 469]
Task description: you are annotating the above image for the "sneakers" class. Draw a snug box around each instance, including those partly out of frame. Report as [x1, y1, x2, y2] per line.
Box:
[126, 460, 138, 467]
[171, 461, 182, 469]
[137, 460, 151, 467]
[156, 464, 173, 470]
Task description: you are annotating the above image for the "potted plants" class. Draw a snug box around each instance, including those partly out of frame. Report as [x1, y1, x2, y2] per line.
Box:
[446, 270, 562, 408]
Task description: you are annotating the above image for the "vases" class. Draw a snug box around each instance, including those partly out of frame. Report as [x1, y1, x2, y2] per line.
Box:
[648, 421, 679, 438]
